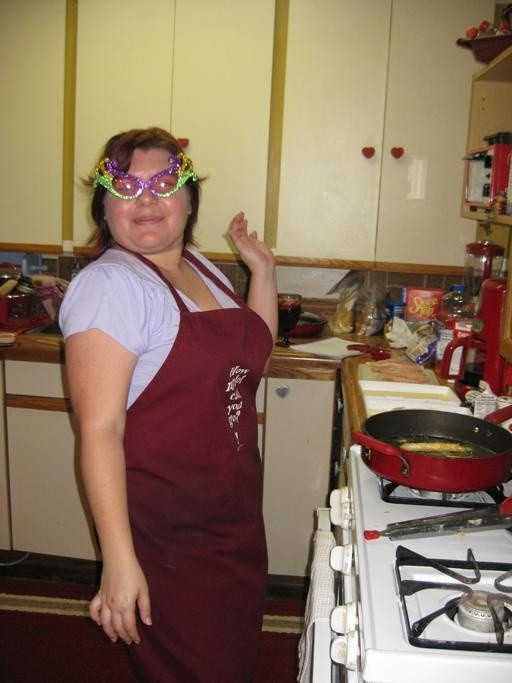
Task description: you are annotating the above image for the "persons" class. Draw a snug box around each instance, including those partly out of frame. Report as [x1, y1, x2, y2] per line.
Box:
[58, 126, 278, 679]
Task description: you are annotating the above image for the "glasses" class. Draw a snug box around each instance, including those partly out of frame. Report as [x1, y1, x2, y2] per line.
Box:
[92, 153, 200, 200]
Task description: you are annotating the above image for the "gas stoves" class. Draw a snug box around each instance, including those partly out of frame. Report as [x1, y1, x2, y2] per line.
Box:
[329, 441, 512, 683]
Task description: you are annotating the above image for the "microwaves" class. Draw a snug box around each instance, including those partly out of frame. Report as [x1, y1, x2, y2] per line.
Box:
[461, 131, 511, 217]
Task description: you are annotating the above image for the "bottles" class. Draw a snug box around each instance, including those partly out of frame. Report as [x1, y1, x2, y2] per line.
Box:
[435, 283, 474, 382]
[466, 18, 509, 38]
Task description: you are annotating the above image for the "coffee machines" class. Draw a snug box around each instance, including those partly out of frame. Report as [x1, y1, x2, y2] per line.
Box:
[441, 276, 512, 400]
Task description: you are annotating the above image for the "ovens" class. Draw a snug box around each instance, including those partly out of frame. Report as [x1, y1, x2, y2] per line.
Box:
[307, 508, 343, 683]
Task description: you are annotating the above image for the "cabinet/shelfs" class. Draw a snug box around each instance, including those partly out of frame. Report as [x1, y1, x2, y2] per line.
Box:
[1, 349, 340, 592]
[1, 0, 471, 279]
[458, 0, 511, 278]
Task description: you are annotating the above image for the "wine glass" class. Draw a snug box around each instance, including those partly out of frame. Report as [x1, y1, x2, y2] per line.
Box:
[275, 292, 301, 346]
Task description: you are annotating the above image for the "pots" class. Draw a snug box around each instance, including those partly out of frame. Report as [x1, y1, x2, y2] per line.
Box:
[455, 37, 509, 63]
[350, 405, 512, 495]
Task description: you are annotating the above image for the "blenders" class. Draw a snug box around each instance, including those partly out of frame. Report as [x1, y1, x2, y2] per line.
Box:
[462, 240, 505, 301]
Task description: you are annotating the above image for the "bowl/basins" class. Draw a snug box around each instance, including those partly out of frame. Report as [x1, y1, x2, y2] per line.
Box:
[288, 310, 327, 337]
[357, 379, 462, 410]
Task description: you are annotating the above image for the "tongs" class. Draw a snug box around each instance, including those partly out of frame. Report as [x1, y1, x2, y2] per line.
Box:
[363, 497, 510, 543]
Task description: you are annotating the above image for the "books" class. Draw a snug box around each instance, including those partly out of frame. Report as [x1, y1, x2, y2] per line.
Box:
[289, 336, 368, 360]
